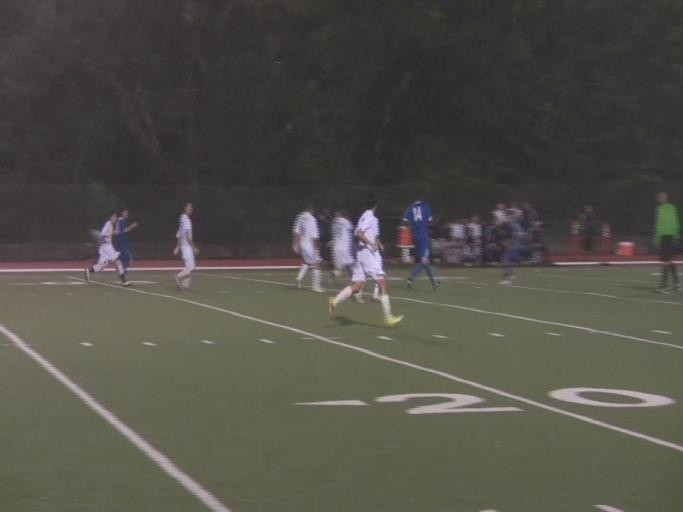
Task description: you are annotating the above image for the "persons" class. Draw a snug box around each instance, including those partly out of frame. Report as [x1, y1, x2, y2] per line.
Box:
[85, 211, 133, 287]
[328, 198, 404, 327]
[112, 206, 139, 285]
[326, 209, 368, 304]
[401, 198, 440, 291]
[349, 229, 383, 303]
[291, 203, 326, 293]
[173, 202, 200, 290]
[435, 201, 601, 285]
[652, 191, 682, 294]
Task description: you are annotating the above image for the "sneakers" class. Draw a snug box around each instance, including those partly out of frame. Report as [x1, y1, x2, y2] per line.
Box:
[174, 274, 181, 290]
[85, 267, 89, 284]
[383, 313, 404, 329]
[122, 280, 133, 286]
[328, 296, 334, 318]
